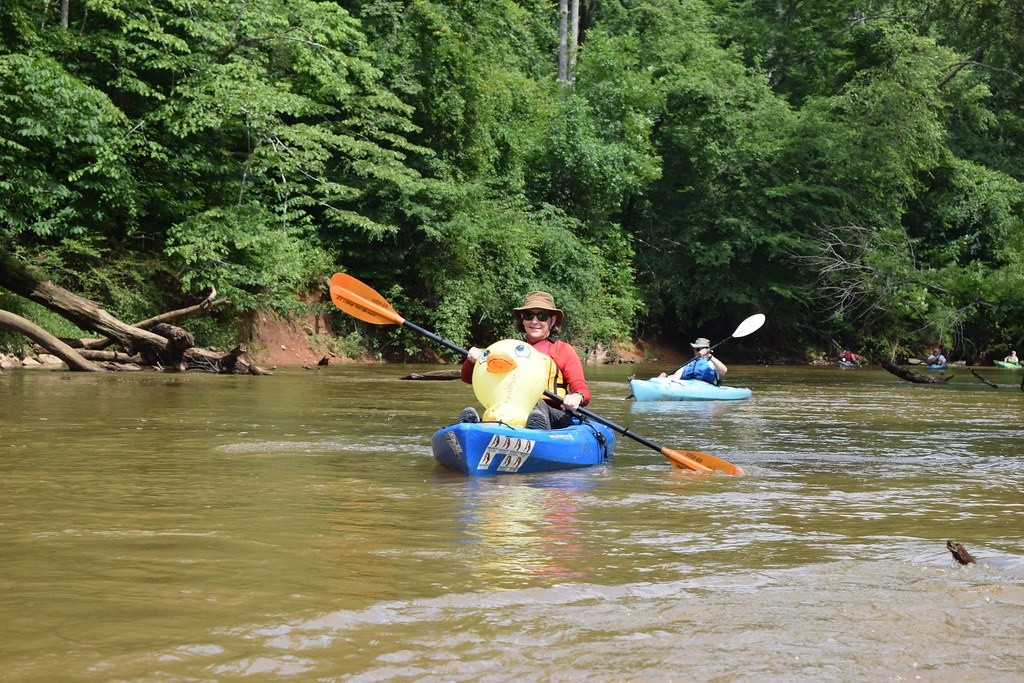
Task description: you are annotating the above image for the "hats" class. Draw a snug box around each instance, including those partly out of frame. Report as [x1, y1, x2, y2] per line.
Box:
[513, 291, 563, 332]
[690, 338, 710, 348]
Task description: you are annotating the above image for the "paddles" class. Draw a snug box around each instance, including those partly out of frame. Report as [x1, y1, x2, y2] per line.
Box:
[624, 313, 766, 400]
[327, 270, 742, 478]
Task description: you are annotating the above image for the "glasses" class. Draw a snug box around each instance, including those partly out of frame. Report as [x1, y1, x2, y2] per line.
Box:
[695, 348, 700, 352]
[523, 311, 551, 321]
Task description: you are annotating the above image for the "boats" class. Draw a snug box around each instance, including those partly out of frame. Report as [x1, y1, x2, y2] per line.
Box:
[430, 415, 617, 476]
[627, 373, 751, 402]
[839, 359, 860, 367]
[929, 363, 949, 370]
[994, 359, 1024, 371]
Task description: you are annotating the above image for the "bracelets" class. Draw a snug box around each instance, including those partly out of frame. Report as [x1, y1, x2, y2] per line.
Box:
[707, 354, 713, 361]
[574, 392, 584, 406]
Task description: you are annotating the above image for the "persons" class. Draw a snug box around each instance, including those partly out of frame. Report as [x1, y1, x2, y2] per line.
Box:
[840, 348, 856, 364]
[1004, 351, 1018, 365]
[658, 338, 728, 386]
[459, 291, 591, 431]
[927, 348, 946, 366]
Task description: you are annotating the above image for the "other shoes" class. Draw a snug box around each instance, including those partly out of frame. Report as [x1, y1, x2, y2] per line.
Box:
[527, 410, 548, 430]
[458, 407, 479, 423]
